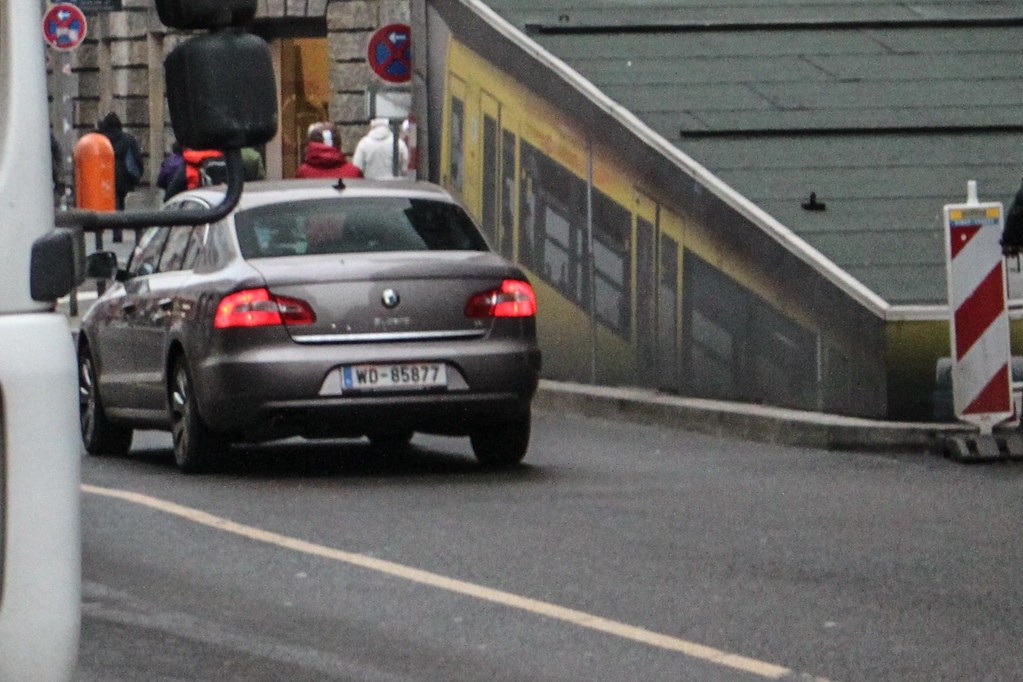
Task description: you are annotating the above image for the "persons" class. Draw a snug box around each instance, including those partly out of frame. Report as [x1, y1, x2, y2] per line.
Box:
[350, 117, 409, 180]
[294, 121, 363, 255]
[98, 112, 143, 246]
[156, 142, 266, 206]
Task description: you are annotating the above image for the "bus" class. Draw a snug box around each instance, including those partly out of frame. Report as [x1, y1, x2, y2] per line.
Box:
[0, 0, 281, 680]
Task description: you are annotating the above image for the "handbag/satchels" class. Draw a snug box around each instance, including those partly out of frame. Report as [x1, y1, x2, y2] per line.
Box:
[123, 134, 142, 186]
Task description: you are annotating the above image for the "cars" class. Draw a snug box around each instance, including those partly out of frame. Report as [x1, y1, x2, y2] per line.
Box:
[75, 176, 544, 467]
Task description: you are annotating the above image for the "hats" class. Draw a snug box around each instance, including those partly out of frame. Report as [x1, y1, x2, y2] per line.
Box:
[94, 109, 125, 131]
[303, 122, 342, 147]
[371, 117, 390, 128]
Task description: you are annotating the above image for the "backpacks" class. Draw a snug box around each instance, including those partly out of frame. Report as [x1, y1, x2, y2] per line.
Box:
[195, 156, 230, 189]
